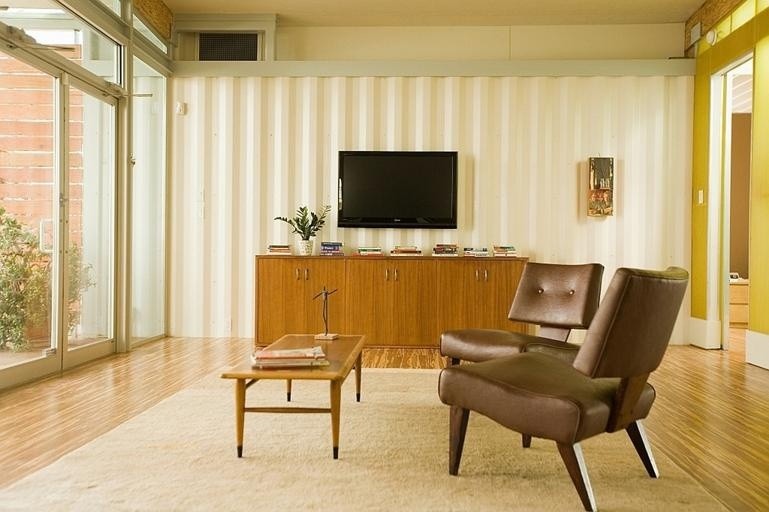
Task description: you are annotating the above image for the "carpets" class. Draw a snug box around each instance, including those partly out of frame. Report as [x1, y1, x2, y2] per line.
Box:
[1, 363, 732, 512]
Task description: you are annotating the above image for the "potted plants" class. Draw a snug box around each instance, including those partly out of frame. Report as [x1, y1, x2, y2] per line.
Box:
[274, 198, 336, 256]
[0, 207, 99, 348]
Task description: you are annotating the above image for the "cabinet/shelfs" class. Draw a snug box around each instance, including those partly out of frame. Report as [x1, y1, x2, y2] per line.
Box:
[436, 253, 532, 349]
[724, 275, 753, 328]
[580, 153, 619, 224]
[253, 251, 348, 349]
[344, 253, 441, 350]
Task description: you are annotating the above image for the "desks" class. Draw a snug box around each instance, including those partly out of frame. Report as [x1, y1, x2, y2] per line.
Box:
[219, 333, 367, 463]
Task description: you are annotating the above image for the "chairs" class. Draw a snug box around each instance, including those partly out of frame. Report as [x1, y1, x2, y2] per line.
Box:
[440, 261, 605, 452]
[438, 262, 694, 512]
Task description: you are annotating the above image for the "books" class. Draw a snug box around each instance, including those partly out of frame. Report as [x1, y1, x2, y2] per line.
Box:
[352, 246, 383, 256]
[431, 243, 460, 257]
[265, 243, 292, 257]
[250, 345, 330, 369]
[389, 245, 423, 257]
[491, 243, 517, 258]
[463, 247, 489, 258]
[319, 240, 345, 257]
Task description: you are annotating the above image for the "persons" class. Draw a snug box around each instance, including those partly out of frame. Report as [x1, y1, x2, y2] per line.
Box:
[311, 284, 339, 337]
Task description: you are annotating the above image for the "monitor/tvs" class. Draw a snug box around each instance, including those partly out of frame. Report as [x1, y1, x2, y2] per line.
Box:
[338, 151, 457, 229]
